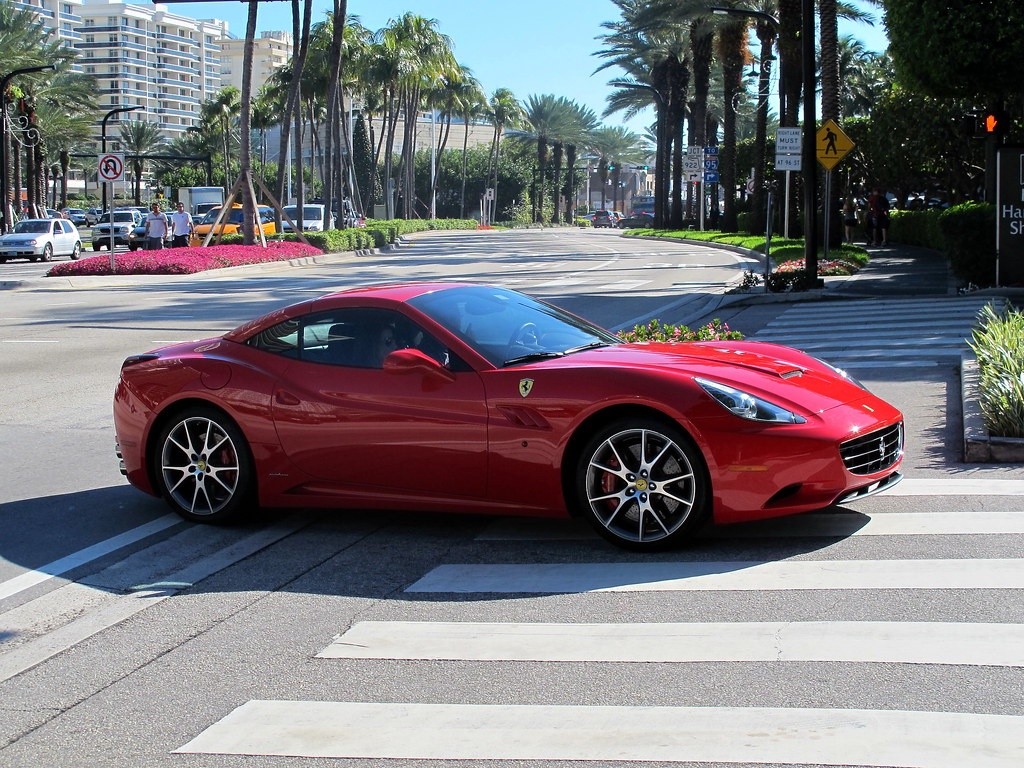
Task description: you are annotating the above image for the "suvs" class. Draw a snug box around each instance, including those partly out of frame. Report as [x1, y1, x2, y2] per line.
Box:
[91, 210, 143, 252]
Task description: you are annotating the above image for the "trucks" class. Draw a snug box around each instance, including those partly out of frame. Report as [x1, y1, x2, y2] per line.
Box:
[177, 187, 224, 212]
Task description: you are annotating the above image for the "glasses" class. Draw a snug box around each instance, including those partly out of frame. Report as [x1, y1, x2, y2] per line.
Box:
[152, 207, 158, 208]
[446, 314, 464, 324]
[177, 206, 184, 208]
[383, 337, 404, 345]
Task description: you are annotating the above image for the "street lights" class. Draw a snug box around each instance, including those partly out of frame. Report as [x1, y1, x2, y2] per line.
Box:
[0, 63, 57, 237]
[100, 105, 147, 215]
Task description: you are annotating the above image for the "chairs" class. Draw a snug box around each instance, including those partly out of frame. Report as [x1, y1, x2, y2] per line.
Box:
[303, 324, 424, 369]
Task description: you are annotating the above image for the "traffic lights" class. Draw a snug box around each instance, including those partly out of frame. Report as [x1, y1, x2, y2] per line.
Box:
[637, 166, 650, 170]
[982, 107, 1004, 142]
[608, 165, 616, 170]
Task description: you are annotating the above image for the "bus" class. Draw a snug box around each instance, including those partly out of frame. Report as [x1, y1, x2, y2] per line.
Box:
[631, 195, 673, 219]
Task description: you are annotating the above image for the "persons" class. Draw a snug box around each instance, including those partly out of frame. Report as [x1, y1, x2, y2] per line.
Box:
[843, 194, 858, 244]
[865, 187, 890, 249]
[172, 202, 194, 247]
[358, 304, 475, 373]
[145, 203, 169, 250]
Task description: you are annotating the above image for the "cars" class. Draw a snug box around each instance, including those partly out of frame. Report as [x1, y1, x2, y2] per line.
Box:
[281, 204, 335, 234]
[61, 208, 86, 226]
[114, 206, 151, 217]
[128, 213, 178, 252]
[46, 208, 63, 219]
[188, 203, 276, 247]
[85, 208, 104, 227]
[0, 218, 83, 262]
[571, 209, 655, 229]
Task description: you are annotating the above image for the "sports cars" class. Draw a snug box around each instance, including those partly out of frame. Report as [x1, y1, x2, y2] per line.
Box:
[108, 277, 908, 552]
